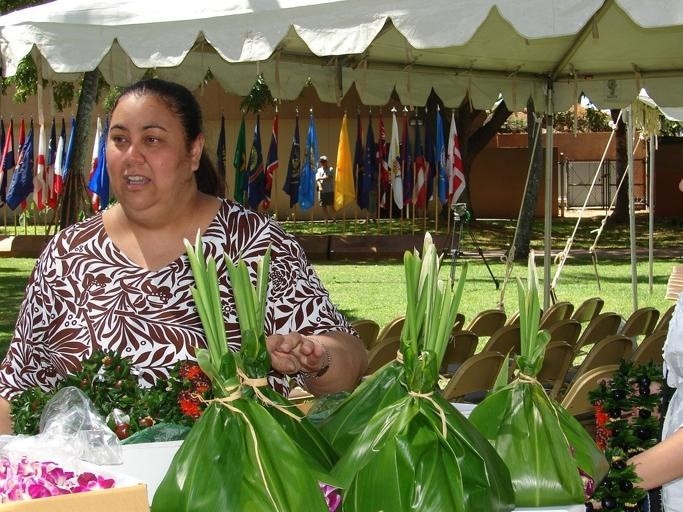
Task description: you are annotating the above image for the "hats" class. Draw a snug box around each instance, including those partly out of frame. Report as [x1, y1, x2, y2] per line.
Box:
[319, 156, 328, 161]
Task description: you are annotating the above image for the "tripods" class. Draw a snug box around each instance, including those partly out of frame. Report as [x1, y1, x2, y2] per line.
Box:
[437, 215, 500, 293]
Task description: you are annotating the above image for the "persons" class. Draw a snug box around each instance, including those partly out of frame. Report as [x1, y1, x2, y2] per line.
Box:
[315, 155, 336, 225]
[587, 178, 683, 512]
[0, 79, 365, 435]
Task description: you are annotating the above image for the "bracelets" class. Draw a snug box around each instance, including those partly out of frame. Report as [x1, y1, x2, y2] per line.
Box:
[300, 334, 330, 380]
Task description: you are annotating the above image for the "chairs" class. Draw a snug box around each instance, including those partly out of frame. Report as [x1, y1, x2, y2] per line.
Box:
[572, 298, 604, 322]
[483, 327, 513, 358]
[351, 321, 380, 349]
[513, 342, 574, 403]
[366, 336, 399, 376]
[539, 301, 574, 328]
[549, 319, 580, 346]
[468, 308, 507, 336]
[621, 307, 659, 338]
[455, 314, 468, 331]
[440, 331, 478, 375]
[380, 318, 407, 341]
[444, 351, 503, 398]
[560, 364, 627, 415]
[632, 331, 667, 370]
[508, 307, 542, 329]
[565, 336, 632, 399]
[564, 312, 620, 383]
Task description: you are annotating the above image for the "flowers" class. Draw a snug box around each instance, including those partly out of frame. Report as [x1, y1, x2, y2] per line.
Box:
[0, 452, 117, 502]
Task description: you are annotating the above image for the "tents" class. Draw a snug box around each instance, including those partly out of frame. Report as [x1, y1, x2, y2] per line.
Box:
[2, 0, 682, 323]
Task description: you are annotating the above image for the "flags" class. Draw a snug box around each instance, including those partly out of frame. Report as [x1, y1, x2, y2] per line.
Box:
[6, 123, 33, 210]
[16, 118, 27, 209]
[262, 113, 280, 208]
[388, 116, 402, 209]
[0, 122, 16, 207]
[232, 117, 247, 204]
[88, 127, 109, 206]
[283, 116, 299, 207]
[424, 115, 434, 208]
[448, 113, 465, 205]
[246, 115, 262, 209]
[378, 114, 387, 208]
[355, 116, 365, 209]
[415, 117, 425, 208]
[366, 115, 375, 193]
[64, 119, 75, 181]
[101, 165, 103, 166]
[47, 124, 58, 208]
[33, 125, 47, 210]
[298, 114, 317, 209]
[86, 117, 103, 212]
[216, 117, 226, 180]
[436, 112, 447, 203]
[54, 124, 65, 192]
[403, 115, 413, 205]
[334, 115, 354, 209]
